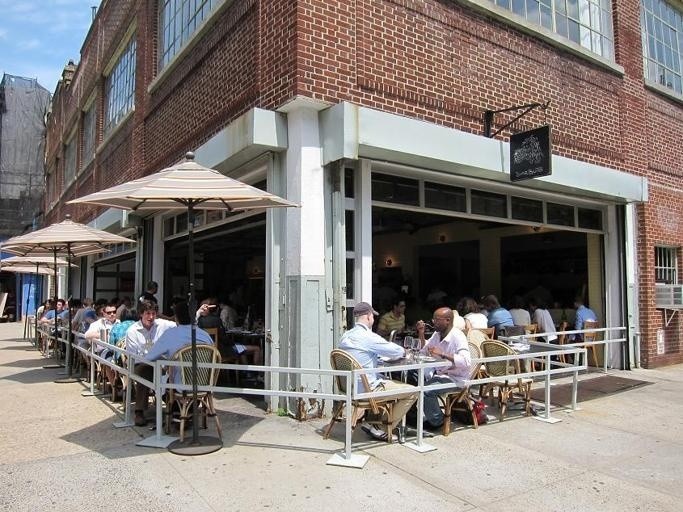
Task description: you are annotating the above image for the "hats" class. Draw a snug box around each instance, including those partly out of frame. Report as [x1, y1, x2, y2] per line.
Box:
[354, 302, 380, 317]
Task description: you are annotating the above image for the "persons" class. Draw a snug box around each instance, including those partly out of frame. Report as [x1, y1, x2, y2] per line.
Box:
[336, 280, 599, 443]
[32, 281, 265, 435]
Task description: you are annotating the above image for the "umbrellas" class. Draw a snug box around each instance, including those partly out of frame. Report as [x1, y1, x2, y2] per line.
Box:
[0, 213, 138, 376]
[62, 150, 302, 447]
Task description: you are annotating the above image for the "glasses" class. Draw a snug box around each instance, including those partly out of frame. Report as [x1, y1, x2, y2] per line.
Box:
[432, 317, 450, 323]
[106, 310, 117, 315]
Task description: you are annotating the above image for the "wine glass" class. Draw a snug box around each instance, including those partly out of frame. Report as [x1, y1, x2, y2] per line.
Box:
[410, 338, 419, 362]
[403, 336, 413, 358]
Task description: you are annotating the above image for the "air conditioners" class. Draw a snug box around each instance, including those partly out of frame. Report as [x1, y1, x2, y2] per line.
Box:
[656, 284, 683, 309]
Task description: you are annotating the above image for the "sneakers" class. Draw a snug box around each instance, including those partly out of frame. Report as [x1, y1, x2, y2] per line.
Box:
[406, 417, 444, 431]
[172, 413, 193, 422]
[135, 415, 146, 425]
[243, 375, 264, 382]
[361, 421, 399, 441]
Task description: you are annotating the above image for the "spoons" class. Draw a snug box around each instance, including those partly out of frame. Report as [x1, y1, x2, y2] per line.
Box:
[420, 321, 436, 330]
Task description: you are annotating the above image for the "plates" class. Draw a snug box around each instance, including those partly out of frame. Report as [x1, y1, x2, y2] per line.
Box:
[422, 358, 436, 362]
[380, 357, 400, 362]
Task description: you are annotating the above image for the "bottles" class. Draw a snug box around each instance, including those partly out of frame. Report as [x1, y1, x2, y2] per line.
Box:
[243, 306, 252, 331]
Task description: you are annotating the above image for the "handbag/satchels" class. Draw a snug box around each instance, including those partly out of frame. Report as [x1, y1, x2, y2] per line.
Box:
[449, 394, 489, 425]
